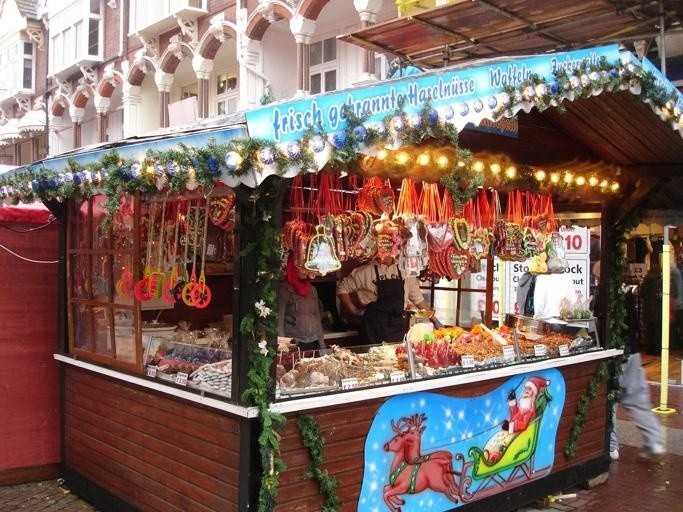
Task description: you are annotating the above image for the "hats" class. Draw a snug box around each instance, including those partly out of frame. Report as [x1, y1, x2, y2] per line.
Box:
[525, 376, 550, 396]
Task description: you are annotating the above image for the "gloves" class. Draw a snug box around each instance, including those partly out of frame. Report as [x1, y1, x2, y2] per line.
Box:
[508, 389, 515, 401]
[502, 420, 509, 431]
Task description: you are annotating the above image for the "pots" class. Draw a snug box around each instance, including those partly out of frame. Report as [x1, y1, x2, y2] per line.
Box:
[141, 318, 178, 356]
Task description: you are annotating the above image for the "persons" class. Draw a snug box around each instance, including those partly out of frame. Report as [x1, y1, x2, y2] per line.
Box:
[277, 255, 327, 350]
[337, 255, 444, 344]
[588, 260, 666, 463]
[640, 261, 663, 357]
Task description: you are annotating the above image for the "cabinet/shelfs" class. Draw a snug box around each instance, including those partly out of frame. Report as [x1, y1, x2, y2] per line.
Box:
[58, 186, 624, 420]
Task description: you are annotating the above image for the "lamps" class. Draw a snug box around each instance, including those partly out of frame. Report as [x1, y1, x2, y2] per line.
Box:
[587, 170, 599, 185]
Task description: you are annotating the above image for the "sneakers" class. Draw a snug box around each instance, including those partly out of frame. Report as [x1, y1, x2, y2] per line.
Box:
[636, 445, 665, 462]
[609, 449, 619, 460]
